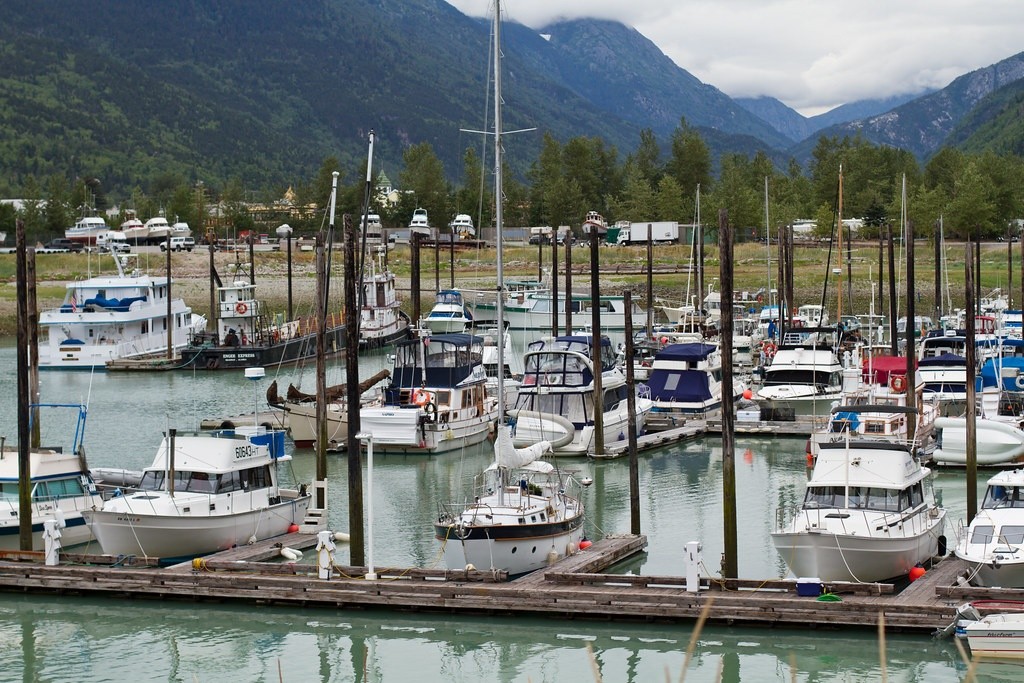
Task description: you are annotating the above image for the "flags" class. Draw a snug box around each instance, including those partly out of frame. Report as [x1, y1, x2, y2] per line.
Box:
[71, 289, 77, 312]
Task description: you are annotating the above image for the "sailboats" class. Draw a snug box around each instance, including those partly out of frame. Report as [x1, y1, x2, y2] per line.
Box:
[433, 1, 593, 573]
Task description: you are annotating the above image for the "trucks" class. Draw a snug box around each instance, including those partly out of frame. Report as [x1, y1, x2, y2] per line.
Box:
[528, 225, 576, 246]
[617, 221, 679, 247]
[96, 230, 131, 253]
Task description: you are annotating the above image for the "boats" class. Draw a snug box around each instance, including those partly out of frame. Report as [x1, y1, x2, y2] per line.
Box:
[64, 184, 112, 243]
[450, 201, 476, 237]
[0, 361, 101, 553]
[581, 210, 609, 235]
[359, 214, 382, 237]
[767, 281, 948, 589]
[354, 244, 412, 352]
[929, 602, 1024, 661]
[180, 224, 347, 369]
[170, 214, 193, 238]
[28, 203, 208, 372]
[119, 217, 150, 245]
[145, 200, 172, 243]
[953, 465, 1024, 587]
[266, 163, 1024, 469]
[79, 366, 314, 568]
[408, 195, 431, 237]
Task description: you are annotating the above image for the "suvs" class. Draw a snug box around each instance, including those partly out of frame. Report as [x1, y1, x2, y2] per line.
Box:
[159, 236, 196, 253]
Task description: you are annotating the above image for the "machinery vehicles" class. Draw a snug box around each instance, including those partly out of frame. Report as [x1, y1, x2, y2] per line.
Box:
[200, 226, 217, 245]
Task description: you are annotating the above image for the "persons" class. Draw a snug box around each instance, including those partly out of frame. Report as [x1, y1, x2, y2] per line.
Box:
[224, 329, 239, 346]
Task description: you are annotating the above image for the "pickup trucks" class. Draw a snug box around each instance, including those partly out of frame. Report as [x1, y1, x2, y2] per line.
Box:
[44, 238, 84, 254]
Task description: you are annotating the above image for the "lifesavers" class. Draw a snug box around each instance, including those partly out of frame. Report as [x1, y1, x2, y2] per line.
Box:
[1015, 375, 1024, 390]
[274, 331, 279, 342]
[413, 389, 431, 405]
[793, 308, 797, 316]
[758, 295, 762, 304]
[891, 376, 906, 392]
[547, 374, 560, 384]
[641, 362, 651, 367]
[764, 344, 778, 356]
[236, 303, 248, 314]
